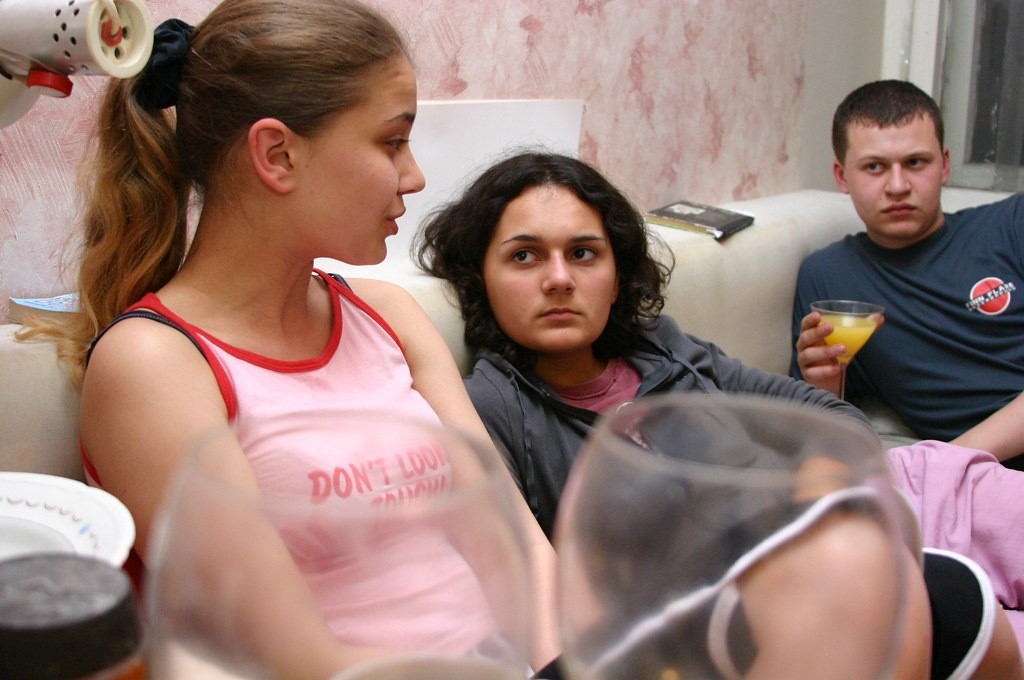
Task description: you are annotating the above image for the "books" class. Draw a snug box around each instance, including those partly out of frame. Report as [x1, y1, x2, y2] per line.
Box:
[646, 201, 755, 241]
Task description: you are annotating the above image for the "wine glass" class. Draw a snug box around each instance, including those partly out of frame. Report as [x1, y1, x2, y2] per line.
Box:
[810, 299, 886, 400]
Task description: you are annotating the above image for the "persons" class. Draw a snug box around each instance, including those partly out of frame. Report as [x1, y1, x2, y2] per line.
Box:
[15, 0, 1024, 680]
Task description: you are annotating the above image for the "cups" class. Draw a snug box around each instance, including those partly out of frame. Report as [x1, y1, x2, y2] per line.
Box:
[553, 395, 910, 680]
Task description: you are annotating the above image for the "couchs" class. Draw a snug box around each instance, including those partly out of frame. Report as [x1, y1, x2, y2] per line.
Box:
[0, 187, 1024, 486]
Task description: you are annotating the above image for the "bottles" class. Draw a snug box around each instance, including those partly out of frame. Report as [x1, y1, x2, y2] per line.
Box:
[0, 551, 153, 680]
